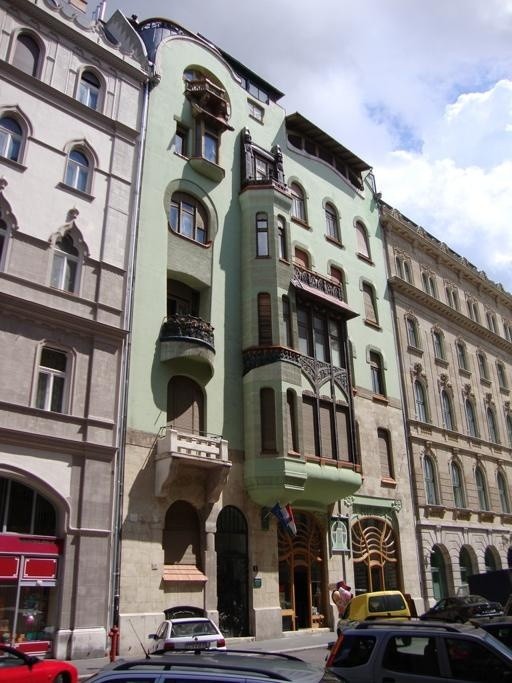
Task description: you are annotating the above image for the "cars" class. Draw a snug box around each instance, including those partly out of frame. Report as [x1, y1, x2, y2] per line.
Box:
[1, 645, 78, 682]
[337, 592, 413, 646]
[147, 617, 228, 657]
[419, 595, 504, 623]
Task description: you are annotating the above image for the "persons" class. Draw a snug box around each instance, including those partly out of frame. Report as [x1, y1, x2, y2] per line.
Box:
[331, 580, 353, 639]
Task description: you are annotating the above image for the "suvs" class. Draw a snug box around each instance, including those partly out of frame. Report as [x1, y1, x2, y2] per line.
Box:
[325, 615, 512, 683]
[81, 648, 341, 683]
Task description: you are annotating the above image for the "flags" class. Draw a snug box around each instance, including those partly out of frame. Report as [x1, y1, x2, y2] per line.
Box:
[282, 503, 297, 537]
[271, 501, 291, 527]
